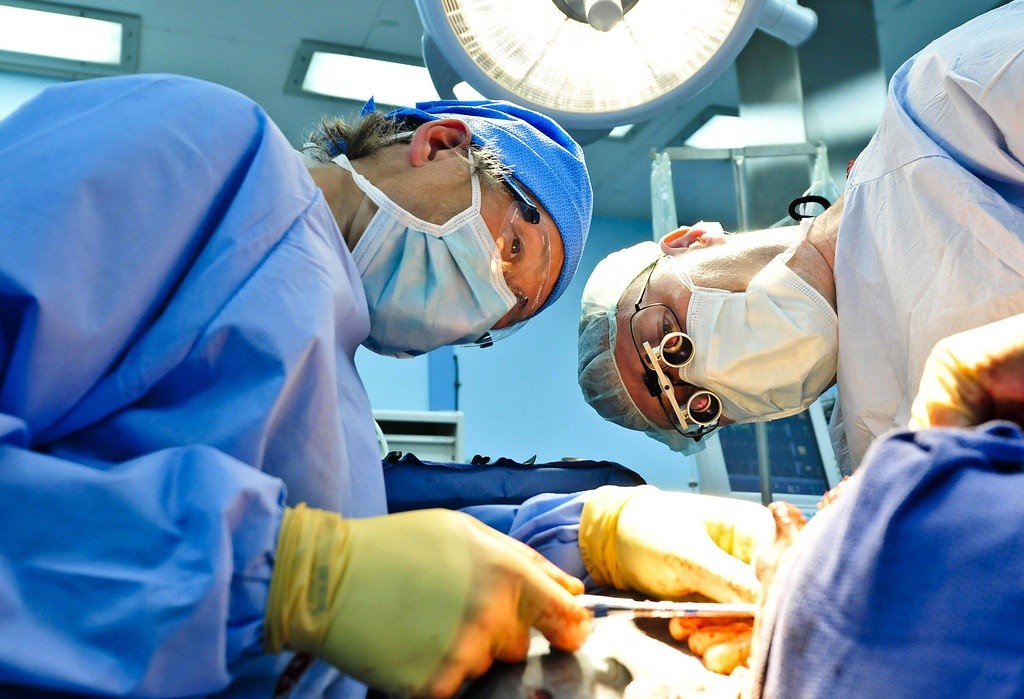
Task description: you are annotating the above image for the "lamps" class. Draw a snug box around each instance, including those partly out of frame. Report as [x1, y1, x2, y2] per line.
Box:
[412, 0, 818, 148]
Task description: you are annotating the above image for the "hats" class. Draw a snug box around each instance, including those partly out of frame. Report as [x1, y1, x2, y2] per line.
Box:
[327, 99, 593, 317]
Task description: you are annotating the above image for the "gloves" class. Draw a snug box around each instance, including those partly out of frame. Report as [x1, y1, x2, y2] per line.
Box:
[267, 502, 591, 699]
[578, 484, 777, 673]
[908, 314, 1024, 430]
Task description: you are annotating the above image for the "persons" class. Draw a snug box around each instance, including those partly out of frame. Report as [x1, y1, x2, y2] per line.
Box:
[0, 70, 782, 698]
[742, 421, 1024, 696]
[574, 0, 1024, 673]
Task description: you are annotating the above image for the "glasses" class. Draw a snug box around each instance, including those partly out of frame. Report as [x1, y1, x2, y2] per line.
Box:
[459, 141, 549, 348]
[628, 259, 721, 442]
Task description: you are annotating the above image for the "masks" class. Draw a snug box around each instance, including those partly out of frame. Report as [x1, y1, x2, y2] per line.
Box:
[665, 244, 840, 427]
[349, 153, 517, 359]
